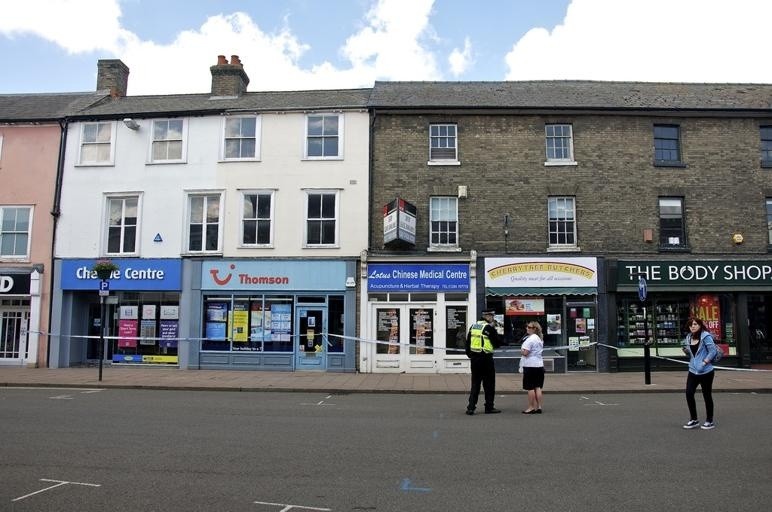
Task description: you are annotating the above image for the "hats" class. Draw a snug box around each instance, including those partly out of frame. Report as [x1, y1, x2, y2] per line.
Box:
[481, 308, 496, 316]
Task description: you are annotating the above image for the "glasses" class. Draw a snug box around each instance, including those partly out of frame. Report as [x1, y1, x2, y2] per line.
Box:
[527, 326, 536, 329]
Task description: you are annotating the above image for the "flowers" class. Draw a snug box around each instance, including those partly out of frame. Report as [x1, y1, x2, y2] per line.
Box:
[92, 257, 119, 272]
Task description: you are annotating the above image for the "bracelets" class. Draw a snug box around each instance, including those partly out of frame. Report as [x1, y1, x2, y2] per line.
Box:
[703, 360, 708, 364]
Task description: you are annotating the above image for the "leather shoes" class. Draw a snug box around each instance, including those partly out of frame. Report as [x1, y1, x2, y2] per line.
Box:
[522, 409, 543, 415]
[465, 410, 475, 415]
[485, 407, 501, 414]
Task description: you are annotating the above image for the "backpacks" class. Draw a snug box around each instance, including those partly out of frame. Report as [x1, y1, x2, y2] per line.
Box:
[712, 342, 723, 364]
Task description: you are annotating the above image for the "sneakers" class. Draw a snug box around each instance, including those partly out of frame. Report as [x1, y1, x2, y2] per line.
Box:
[701, 422, 715, 430]
[684, 420, 700, 429]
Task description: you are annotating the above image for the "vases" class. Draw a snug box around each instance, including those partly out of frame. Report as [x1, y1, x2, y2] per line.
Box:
[97, 269, 111, 279]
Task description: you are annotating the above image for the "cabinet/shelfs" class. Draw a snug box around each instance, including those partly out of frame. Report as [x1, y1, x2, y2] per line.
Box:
[617, 303, 680, 345]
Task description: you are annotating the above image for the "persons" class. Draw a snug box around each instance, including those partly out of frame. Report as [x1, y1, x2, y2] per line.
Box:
[509, 301, 524, 312]
[680, 316, 720, 429]
[463, 307, 503, 418]
[516, 319, 548, 415]
